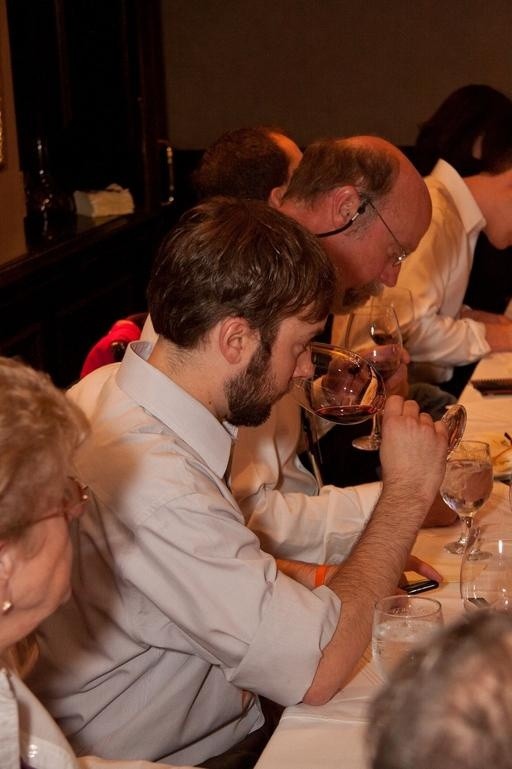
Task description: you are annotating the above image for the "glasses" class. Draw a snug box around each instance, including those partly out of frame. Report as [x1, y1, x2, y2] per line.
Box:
[357, 192, 408, 267]
[0, 474, 89, 538]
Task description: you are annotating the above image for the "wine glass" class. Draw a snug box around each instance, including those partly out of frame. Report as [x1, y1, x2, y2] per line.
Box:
[439, 437, 493, 557]
[287, 288, 468, 457]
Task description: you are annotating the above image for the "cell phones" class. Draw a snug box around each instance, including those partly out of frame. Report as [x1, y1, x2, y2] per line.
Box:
[402, 569, 440, 594]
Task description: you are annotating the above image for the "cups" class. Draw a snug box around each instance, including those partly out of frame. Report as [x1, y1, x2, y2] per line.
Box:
[372, 525, 511, 687]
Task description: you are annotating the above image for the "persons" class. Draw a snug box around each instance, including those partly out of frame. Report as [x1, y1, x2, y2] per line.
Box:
[0, 84, 512, 769]
